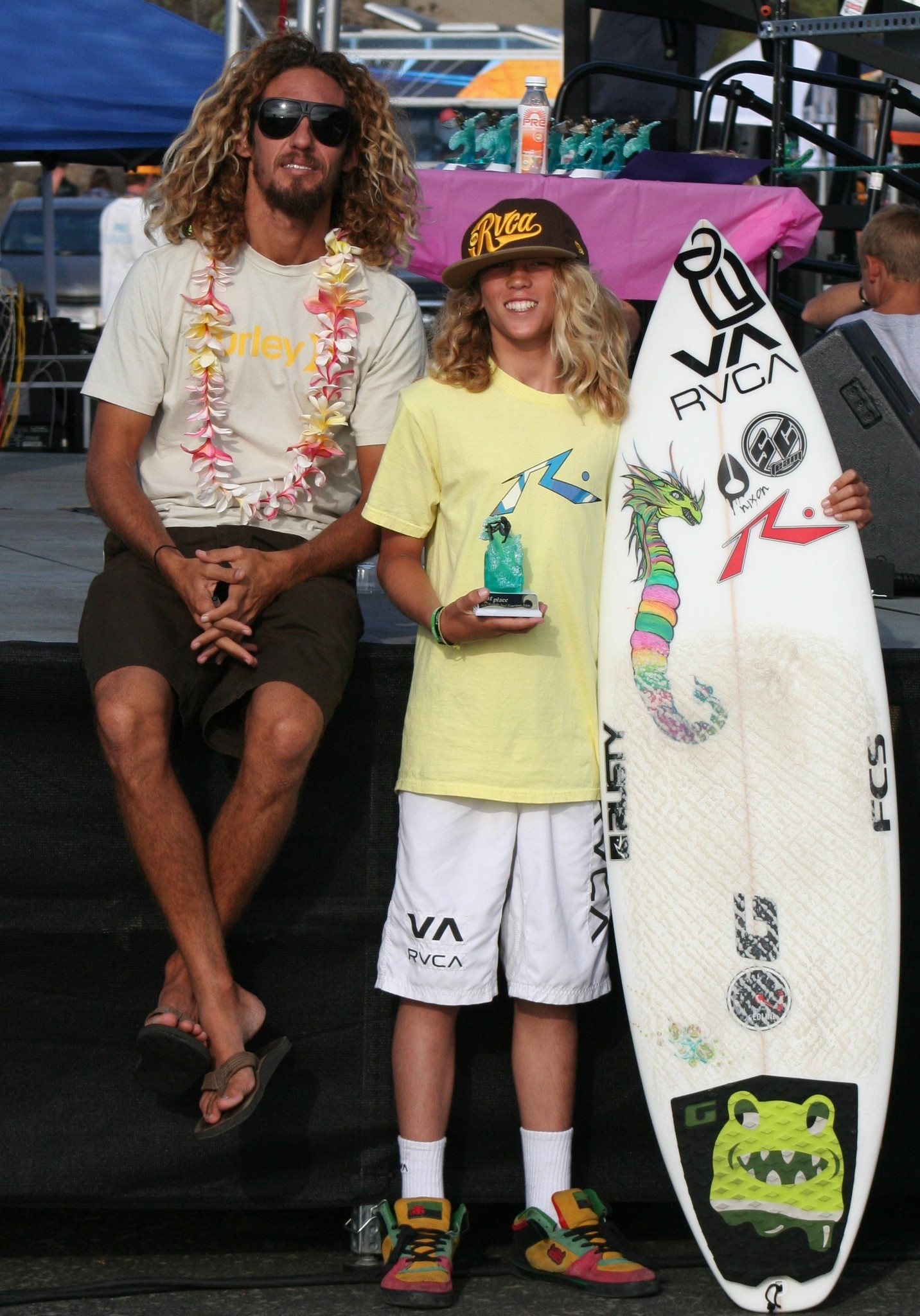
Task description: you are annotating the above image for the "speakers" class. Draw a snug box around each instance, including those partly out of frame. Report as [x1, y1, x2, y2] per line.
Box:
[798, 320, 920, 594]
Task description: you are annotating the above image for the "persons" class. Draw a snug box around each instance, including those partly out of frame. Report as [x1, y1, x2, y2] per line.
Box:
[0, 164, 171, 327]
[801, 282, 870, 330]
[826, 204, 920, 404]
[81, 32, 431, 1139]
[362, 198, 875, 1308]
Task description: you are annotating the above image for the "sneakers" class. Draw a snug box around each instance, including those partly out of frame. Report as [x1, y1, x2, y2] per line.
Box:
[375, 1195, 467, 1309]
[512, 1187, 658, 1300]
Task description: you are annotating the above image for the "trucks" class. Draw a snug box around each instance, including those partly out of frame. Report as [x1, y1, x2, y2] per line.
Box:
[338, 2, 562, 163]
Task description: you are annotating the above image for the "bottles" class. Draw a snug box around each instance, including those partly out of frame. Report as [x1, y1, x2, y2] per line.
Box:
[515, 76, 551, 175]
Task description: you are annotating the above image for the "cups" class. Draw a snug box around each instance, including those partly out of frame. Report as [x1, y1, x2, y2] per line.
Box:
[356, 562, 384, 594]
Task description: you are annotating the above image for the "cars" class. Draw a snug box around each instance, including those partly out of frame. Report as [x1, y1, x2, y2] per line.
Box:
[0, 192, 119, 330]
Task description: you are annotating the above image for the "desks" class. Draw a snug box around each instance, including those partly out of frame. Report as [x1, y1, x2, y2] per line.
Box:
[394, 165, 824, 305]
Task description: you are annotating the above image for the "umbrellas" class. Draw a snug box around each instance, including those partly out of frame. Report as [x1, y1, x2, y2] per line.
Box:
[0, 0, 251, 317]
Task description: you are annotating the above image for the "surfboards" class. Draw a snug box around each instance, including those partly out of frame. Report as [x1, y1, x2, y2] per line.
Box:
[598, 218, 902, 1315]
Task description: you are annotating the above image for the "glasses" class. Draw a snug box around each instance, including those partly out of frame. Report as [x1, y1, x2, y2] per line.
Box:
[253, 98, 356, 147]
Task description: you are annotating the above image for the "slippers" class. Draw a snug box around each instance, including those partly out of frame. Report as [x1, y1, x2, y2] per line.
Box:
[136, 1011, 213, 1093]
[194, 1034, 291, 1140]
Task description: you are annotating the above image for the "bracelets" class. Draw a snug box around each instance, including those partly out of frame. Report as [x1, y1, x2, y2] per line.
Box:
[431, 606, 463, 648]
[859, 285, 870, 306]
[154, 545, 182, 574]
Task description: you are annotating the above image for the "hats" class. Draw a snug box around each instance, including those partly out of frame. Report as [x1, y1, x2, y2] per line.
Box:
[443, 198, 589, 288]
[124, 148, 163, 178]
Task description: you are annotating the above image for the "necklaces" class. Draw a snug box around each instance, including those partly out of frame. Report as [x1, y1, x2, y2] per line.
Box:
[179, 227, 370, 527]
